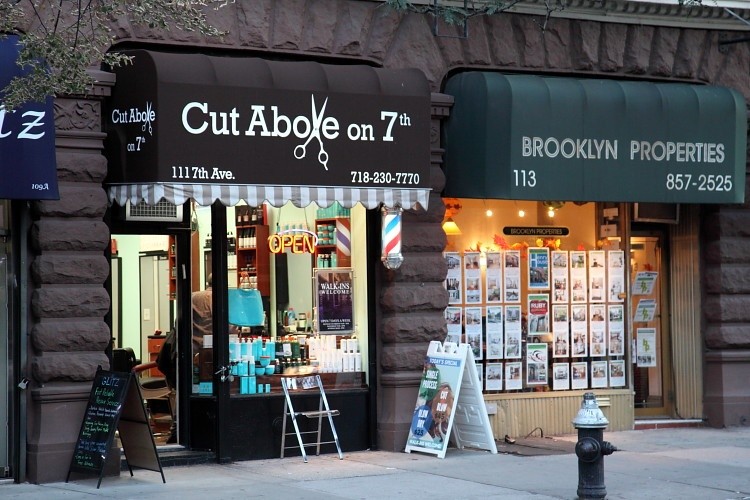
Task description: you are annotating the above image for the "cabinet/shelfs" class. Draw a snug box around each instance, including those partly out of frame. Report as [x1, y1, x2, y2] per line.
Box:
[168, 230, 200, 301]
[236, 225, 270, 297]
[315, 218, 351, 269]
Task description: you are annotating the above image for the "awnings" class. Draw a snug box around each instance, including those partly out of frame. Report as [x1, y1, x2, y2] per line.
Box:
[440, 69, 749, 206]
[103, 49, 435, 213]
[0, 34, 60, 200]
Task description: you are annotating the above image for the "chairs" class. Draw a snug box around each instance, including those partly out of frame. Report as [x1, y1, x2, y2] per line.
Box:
[111, 347, 176, 427]
[281, 375, 345, 464]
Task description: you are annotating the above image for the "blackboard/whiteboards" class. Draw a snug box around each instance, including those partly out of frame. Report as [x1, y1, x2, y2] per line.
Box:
[66, 364, 167, 489]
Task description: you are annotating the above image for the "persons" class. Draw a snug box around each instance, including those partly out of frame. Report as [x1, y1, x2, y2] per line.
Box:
[191, 272, 212, 356]
[155, 318, 177, 444]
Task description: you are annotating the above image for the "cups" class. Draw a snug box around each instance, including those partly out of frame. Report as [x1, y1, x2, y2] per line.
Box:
[240, 276, 257, 291]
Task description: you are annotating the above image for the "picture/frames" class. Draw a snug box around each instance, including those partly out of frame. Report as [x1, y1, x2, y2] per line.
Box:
[315, 269, 356, 335]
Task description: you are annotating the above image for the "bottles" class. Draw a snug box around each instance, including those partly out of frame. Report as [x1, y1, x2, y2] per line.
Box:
[237, 208, 243, 224]
[171, 264, 176, 277]
[204, 234, 212, 249]
[252, 208, 256, 224]
[238, 229, 256, 248]
[226, 231, 235, 247]
[256, 206, 263, 224]
[282, 308, 298, 326]
[171, 243, 175, 255]
[243, 209, 250, 225]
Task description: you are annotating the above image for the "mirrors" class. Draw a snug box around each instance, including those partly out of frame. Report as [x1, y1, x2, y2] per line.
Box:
[111, 256, 123, 350]
[139, 250, 173, 378]
